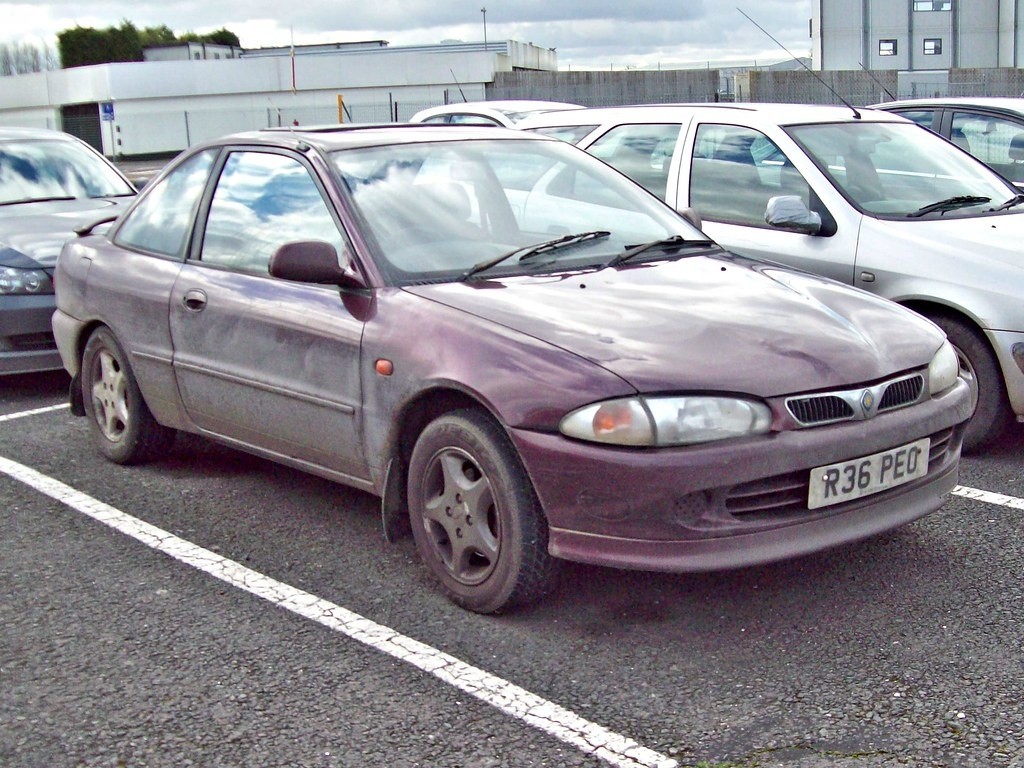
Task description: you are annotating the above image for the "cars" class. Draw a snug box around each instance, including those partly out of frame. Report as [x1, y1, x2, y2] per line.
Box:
[0, 126, 150, 378]
[50, 5, 1024, 615]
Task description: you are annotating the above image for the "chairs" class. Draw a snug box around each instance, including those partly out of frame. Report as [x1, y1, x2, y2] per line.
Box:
[781, 149, 882, 216]
[409, 181, 487, 249]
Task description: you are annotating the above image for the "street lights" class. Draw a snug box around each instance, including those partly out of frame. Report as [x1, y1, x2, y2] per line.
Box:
[480, 7, 487, 49]
